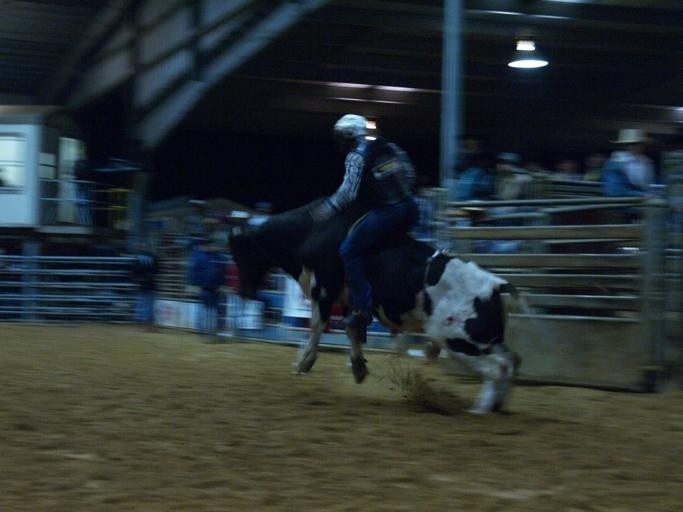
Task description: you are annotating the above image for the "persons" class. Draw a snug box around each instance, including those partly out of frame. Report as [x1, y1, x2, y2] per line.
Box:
[311, 114, 421, 329]
[130, 242, 160, 322]
[188, 236, 226, 308]
[452, 129, 654, 253]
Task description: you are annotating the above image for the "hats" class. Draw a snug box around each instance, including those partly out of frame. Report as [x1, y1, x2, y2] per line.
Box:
[608, 129, 650, 143]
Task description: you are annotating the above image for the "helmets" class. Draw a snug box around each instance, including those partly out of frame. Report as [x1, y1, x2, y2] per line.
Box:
[337, 114, 368, 136]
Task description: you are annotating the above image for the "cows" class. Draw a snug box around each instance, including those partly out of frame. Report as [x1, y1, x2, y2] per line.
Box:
[227, 209, 534, 415]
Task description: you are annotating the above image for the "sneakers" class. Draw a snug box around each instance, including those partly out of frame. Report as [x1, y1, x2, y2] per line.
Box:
[344, 310, 372, 326]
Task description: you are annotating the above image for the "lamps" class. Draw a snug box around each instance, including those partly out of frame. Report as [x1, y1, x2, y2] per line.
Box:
[506, 39, 551, 70]
